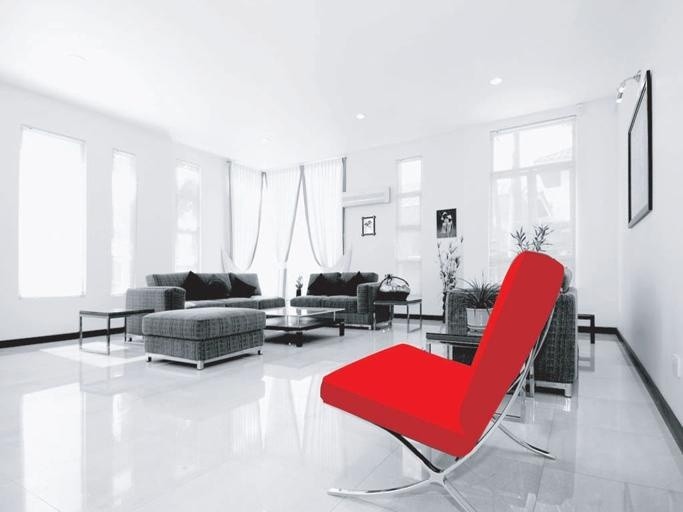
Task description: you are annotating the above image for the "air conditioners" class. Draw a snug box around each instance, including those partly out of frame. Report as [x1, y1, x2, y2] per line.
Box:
[337, 186, 391, 210]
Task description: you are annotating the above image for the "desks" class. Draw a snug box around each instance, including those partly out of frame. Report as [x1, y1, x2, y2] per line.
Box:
[373, 298, 423, 334]
[78, 307, 154, 353]
[424, 328, 536, 402]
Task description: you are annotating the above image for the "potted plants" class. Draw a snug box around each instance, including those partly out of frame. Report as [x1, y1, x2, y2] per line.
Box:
[438, 237, 462, 324]
[448, 274, 503, 335]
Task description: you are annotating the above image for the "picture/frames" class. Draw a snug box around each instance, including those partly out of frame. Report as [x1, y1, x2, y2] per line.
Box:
[625, 70, 652, 228]
[434, 207, 458, 240]
[360, 215, 377, 237]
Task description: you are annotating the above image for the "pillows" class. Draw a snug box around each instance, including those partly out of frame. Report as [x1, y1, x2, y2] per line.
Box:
[327, 277, 347, 295]
[181, 269, 209, 301]
[229, 272, 258, 299]
[307, 273, 332, 294]
[207, 272, 230, 299]
[343, 270, 364, 295]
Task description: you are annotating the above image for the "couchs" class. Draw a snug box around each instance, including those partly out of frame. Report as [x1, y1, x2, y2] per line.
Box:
[142, 306, 265, 370]
[290, 272, 393, 331]
[124, 271, 285, 343]
[443, 268, 580, 400]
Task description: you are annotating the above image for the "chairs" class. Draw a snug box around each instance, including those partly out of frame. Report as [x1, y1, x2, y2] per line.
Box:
[319, 250, 567, 512]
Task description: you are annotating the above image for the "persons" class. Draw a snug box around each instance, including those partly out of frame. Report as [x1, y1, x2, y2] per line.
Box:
[440, 212, 453, 238]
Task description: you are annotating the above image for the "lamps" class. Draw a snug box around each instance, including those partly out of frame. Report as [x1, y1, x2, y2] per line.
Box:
[613, 68, 642, 102]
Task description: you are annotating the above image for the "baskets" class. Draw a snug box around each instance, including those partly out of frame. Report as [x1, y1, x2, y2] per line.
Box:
[377, 276, 410, 300]
